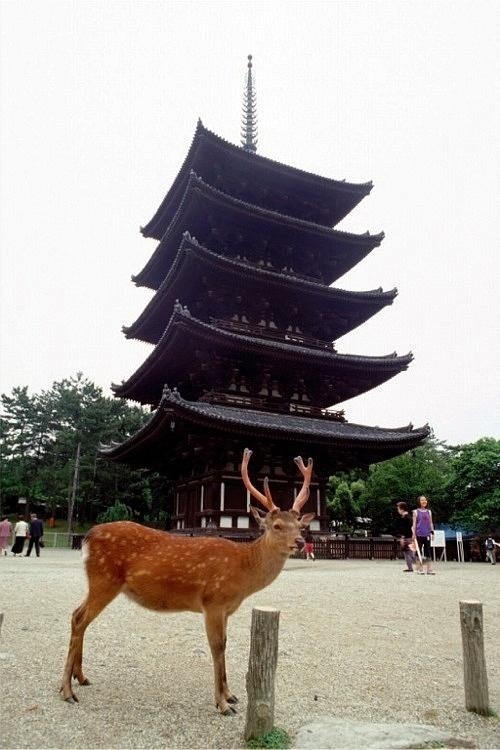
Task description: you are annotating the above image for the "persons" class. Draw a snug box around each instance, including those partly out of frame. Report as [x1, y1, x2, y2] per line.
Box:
[303, 530, 315, 561]
[396, 502, 416, 571]
[484, 534, 497, 564]
[411, 495, 436, 575]
[23, 513, 43, 558]
[11, 514, 30, 557]
[0, 514, 13, 556]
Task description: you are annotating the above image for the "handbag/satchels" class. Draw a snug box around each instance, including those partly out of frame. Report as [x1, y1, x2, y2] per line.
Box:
[39, 541, 45, 548]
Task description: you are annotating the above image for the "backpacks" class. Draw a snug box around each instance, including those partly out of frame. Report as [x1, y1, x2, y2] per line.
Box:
[486, 539, 494, 551]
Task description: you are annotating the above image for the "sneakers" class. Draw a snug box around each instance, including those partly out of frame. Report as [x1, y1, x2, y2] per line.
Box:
[403, 566, 436, 576]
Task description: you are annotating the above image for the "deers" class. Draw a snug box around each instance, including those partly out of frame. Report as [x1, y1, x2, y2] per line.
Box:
[59, 448, 316, 717]
